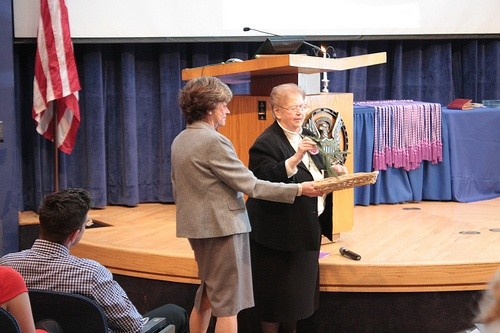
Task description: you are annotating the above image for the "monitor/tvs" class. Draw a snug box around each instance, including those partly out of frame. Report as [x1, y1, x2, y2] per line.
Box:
[252, 36, 307, 59]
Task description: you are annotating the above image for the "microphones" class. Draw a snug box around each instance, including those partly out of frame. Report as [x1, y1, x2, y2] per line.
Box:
[340, 247, 361, 260]
[244, 27, 326, 58]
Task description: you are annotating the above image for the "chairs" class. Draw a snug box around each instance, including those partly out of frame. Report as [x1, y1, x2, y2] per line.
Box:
[27, 289, 175, 333]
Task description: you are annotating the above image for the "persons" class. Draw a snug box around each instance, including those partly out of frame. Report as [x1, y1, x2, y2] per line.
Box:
[169, 76, 323, 333]
[0, 266, 63, 333]
[245, 83, 348, 333]
[0, 187, 191, 333]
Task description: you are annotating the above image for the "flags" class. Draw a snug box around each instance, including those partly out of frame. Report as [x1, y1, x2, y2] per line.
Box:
[32, 0, 82, 155]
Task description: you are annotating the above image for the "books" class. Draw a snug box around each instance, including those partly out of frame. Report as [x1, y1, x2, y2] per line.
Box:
[448, 98, 473, 110]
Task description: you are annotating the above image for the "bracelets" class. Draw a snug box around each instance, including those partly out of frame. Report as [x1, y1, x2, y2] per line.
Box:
[297, 184, 303, 195]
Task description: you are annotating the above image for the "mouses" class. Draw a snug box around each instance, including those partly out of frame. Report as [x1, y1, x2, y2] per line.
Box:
[225, 58, 243, 63]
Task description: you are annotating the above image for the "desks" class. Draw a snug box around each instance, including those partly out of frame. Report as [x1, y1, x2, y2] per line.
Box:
[353, 100, 500, 205]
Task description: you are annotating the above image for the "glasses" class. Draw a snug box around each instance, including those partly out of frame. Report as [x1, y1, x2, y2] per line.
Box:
[278, 105, 306, 114]
[85, 216, 94, 227]
